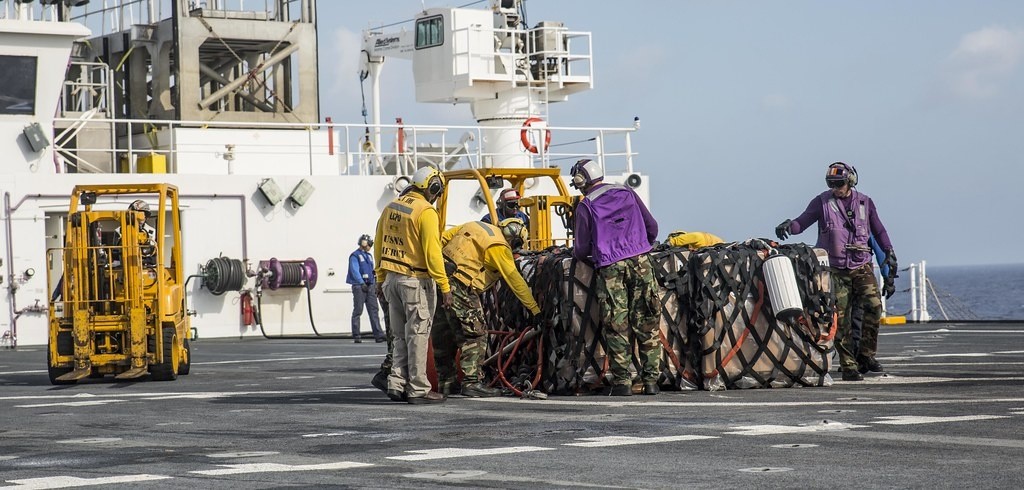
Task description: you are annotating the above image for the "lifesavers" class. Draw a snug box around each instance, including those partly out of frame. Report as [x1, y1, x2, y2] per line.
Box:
[521, 118, 551, 153]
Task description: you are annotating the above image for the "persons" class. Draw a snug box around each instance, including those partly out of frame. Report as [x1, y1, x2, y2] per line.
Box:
[776, 162, 898, 381]
[570, 158, 660, 396]
[431, 218, 545, 396]
[346, 235, 386, 343]
[113, 200, 157, 265]
[371, 166, 453, 404]
[481, 188, 530, 231]
[658, 230, 723, 250]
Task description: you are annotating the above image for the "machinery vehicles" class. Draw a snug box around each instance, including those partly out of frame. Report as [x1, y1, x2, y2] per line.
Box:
[48, 184, 198, 387]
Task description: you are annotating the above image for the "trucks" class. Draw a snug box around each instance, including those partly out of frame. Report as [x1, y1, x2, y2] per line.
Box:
[425, 165, 837, 400]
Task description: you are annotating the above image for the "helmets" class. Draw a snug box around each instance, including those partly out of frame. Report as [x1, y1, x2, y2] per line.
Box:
[569, 159, 605, 196]
[358, 234, 375, 248]
[128, 200, 152, 217]
[826, 163, 858, 187]
[499, 218, 528, 250]
[411, 166, 446, 205]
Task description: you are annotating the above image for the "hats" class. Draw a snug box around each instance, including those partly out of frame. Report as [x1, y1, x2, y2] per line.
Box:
[497, 188, 520, 203]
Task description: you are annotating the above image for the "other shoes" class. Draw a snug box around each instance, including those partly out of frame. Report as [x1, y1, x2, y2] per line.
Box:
[375, 335, 387, 344]
[354, 336, 361, 343]
[641, 385, 660, 394]
[842, 370, 865, 382]
[605, 385, 633, 396]
[462, 383, 502, 397]
[372, 372, 390, 395]
[438, 378, 461, 394]
[390, 391, 407, 402]
[409, 391, 447, 404]
[858, 356, 883, 372]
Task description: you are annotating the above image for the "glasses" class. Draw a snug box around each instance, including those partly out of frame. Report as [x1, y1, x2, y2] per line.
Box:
[827, 180, 847, 188]
[503, 201, 519, 210]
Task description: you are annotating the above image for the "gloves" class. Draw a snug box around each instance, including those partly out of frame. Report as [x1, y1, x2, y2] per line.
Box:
[882, 279, 896, 300]
[880, 250, 898, 279]
[775, 218, 794, 240]
[362, 285, 369, 293]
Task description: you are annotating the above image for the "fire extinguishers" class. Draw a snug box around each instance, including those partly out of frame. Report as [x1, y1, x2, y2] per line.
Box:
[243, 288, 254, 324]
